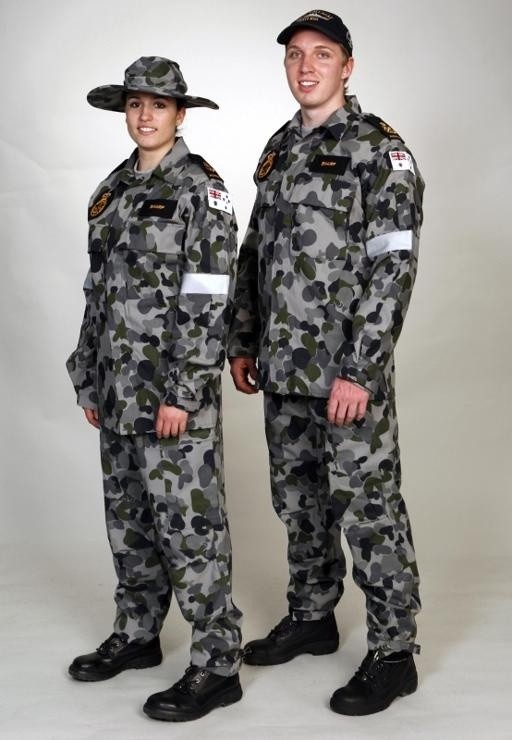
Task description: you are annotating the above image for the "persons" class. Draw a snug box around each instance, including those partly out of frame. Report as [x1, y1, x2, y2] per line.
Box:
[224, 6, 429, 718]
[63, 55, 244, 724]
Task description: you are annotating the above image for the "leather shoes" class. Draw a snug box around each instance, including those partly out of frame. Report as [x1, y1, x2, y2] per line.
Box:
[67, 632, 163, 682]
[330, 648, 419, 716]
[244, 613, 341, 667]
[142, 664, 242, 721]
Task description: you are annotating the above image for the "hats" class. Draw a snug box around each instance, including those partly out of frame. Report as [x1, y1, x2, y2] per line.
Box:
[86, 55, 219, 112]
[276, 9, 353, 59]
